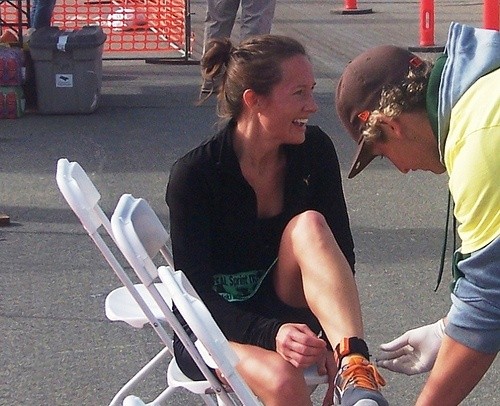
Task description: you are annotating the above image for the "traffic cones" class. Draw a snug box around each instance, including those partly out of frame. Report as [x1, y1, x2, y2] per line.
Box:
[407, 0, 445, 52]
[483, 0, 500, 32]
[329, 0, 373, 15]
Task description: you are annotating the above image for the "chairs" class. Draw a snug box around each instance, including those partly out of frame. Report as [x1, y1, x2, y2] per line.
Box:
[53, 157, 174, 406]
[158, 265, 267, 405]
[109, 193, 330, 405]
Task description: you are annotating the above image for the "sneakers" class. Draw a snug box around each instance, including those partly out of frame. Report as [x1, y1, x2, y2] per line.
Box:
[332, 356, 389, 406]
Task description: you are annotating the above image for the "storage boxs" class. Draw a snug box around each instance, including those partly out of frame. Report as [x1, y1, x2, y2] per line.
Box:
[0, 48, 27, 87]
[1, 88, 26, 119]
[29, 23, 104, 113]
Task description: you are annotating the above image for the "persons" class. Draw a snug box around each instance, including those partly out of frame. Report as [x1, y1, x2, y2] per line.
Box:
[335, 23, 500, 406]
[165, 34, 390, 406]
[200, 1, 276, 92]
[27, 0, 54, 34]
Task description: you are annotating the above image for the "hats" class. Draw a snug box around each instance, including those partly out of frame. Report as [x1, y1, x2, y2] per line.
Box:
[335, 46, 424, 179]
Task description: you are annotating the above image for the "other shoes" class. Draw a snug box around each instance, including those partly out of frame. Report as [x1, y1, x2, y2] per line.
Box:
[202, 79, 218, 92]
